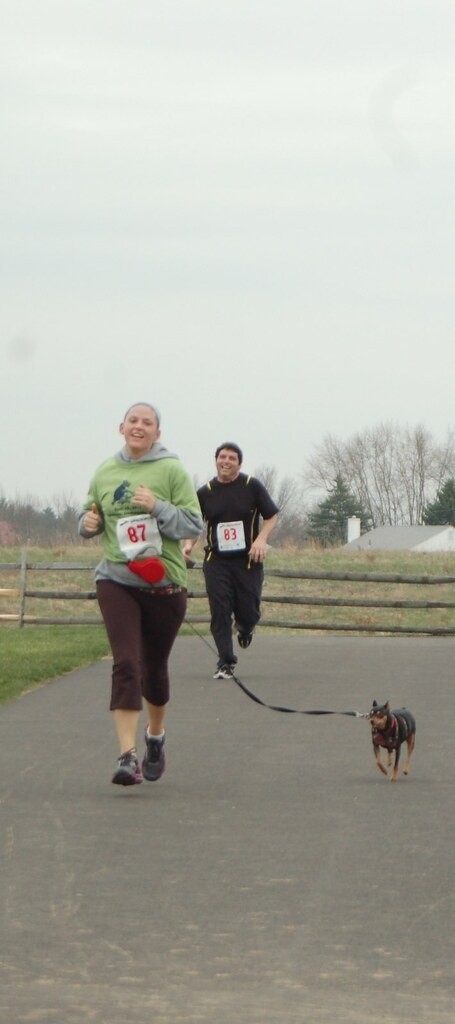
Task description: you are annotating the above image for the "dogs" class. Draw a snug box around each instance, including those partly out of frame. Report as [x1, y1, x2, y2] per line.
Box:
[368, 699, 417, 784]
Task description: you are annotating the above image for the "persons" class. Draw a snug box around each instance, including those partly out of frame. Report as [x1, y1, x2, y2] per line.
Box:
[182, 442, 279, 679]
[78, 403, 203, 785]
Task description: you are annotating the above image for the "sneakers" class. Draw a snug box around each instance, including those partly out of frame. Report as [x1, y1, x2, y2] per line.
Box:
[141, 723, 165, 781]
[213, 663, 236, 679]
[236, 631, 253, 649]
[112, 747, 144, 785]
